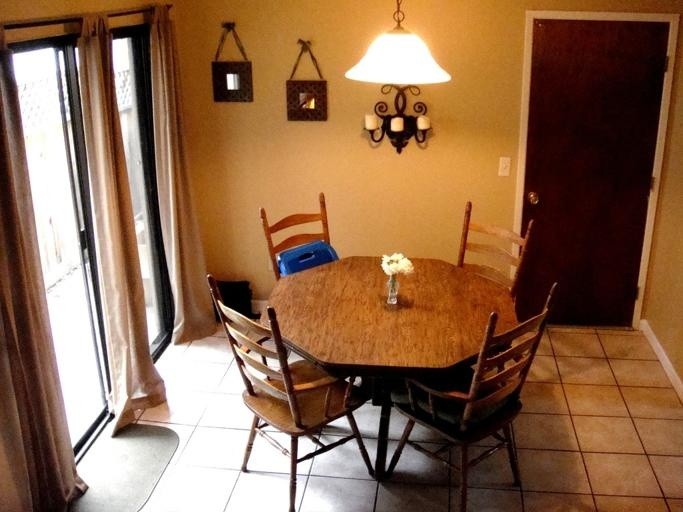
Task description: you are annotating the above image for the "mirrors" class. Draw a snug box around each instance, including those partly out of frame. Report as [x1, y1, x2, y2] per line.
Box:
[286, 79, 328, 123]
[212, 60, 254, 103]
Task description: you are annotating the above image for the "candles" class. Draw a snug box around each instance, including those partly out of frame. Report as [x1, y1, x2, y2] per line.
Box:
[362, 114, 431, 134]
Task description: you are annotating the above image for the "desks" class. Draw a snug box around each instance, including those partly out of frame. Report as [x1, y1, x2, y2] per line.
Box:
[263, 254, 522, 483]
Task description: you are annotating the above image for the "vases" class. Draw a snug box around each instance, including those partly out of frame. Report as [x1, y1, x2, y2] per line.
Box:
[385, 275, 401, 307]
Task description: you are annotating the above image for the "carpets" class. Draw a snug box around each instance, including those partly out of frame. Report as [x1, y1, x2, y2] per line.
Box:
[67, 417, 181, 512]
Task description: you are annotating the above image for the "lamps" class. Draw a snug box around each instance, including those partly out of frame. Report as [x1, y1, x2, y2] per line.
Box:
[345, 1, 453, 85]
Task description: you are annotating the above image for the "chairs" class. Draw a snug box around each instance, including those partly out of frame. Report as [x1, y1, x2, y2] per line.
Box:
[382, 281, 559, 510]
[205, 272, 374, 510]
[455, 201, 536, 297]
[258, 191, 332, 283]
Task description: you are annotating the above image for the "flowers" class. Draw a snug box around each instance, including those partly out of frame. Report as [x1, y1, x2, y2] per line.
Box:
[380, 251, 417, 275]
